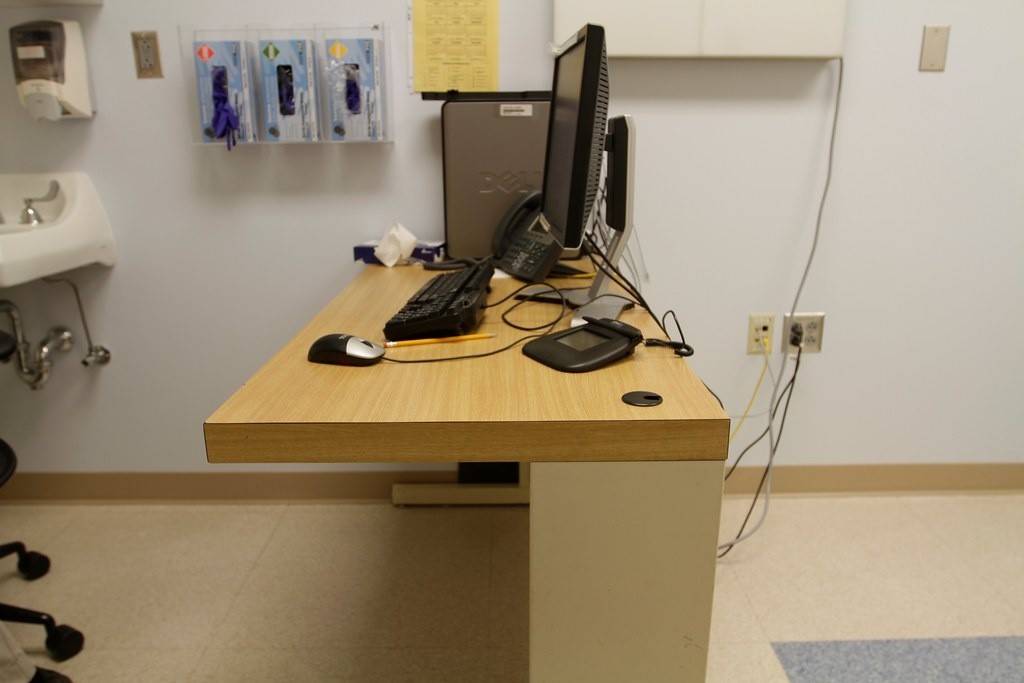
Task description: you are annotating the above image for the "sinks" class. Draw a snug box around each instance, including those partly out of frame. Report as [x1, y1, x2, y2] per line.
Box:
[0, 171, 117, 288]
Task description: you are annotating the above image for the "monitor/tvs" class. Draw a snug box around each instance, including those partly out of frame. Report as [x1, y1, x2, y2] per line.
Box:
[515, 22, 635, 328]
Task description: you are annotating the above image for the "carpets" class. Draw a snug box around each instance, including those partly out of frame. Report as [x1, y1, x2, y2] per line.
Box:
[772, 637, 1024, 682]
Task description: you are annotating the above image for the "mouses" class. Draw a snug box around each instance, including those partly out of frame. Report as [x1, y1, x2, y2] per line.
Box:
[307, 333, 386, 366]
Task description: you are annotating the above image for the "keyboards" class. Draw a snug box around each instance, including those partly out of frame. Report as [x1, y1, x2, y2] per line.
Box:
[383, 261, 495, 342]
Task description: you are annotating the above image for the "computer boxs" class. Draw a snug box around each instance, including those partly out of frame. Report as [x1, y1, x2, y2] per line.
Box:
[441, 90, 583, 262]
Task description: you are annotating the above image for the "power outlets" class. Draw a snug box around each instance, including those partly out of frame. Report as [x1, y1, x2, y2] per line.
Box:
[781, 312, 825, 353]
[131, 31, 163, 79]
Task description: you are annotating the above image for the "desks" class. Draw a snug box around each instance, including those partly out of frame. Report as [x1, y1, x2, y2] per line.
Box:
[203, 245, 730, 683]
